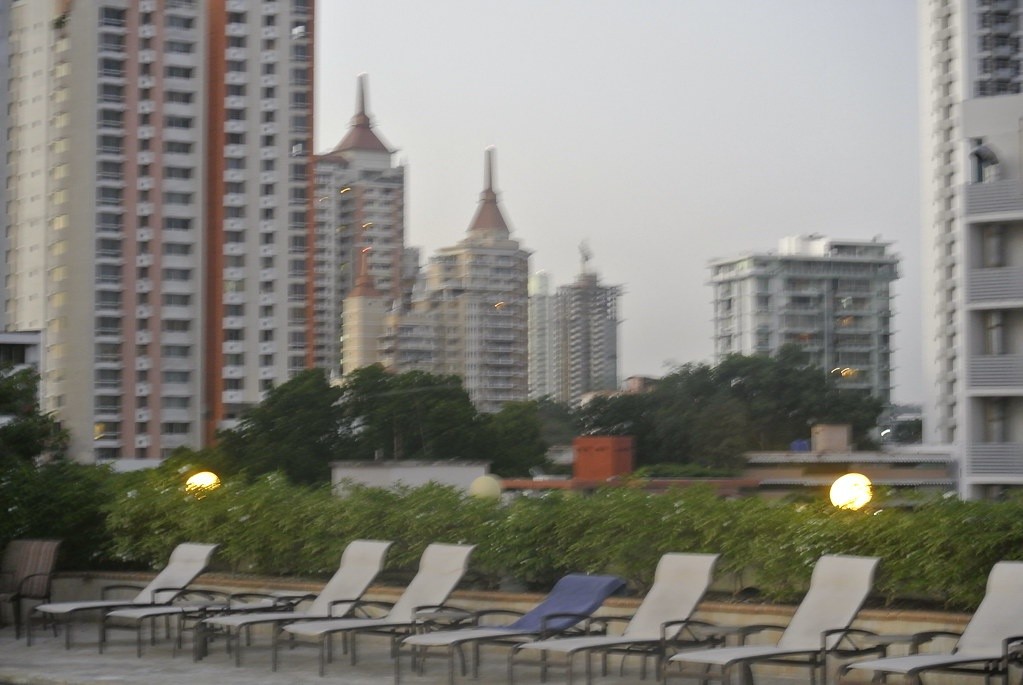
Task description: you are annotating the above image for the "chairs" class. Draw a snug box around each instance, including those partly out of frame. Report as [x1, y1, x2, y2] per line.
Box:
[0, 539, 1023, 685]
